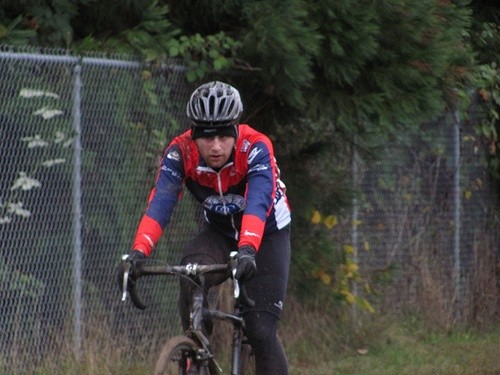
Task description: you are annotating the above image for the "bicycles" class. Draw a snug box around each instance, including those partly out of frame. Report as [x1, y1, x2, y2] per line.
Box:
[120, 251, 260, 375]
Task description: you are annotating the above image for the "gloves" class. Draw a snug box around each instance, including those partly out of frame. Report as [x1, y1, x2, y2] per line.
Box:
[226, 245, 257, 282]
[118, 250, 146, 292]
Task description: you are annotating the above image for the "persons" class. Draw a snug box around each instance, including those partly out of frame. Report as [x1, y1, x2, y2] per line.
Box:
[121, 79, 294, 375]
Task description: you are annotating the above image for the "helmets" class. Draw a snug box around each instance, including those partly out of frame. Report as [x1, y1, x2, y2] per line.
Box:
[186, 81, 243, 129]
